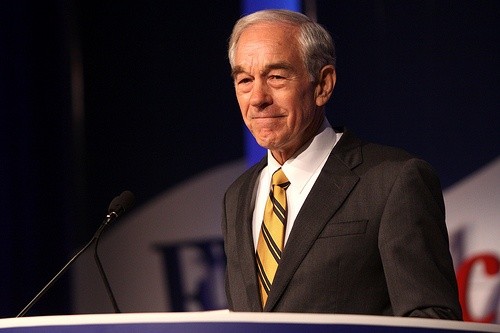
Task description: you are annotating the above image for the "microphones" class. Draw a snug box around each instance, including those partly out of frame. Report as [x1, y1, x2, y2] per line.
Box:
[16, 190, 136, 317]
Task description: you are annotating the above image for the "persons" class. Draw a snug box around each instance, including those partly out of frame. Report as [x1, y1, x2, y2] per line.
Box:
[220, 9, 464, 322]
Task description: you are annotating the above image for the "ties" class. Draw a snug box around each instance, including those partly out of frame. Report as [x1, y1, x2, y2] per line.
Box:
[255, 167, 291, 311]
